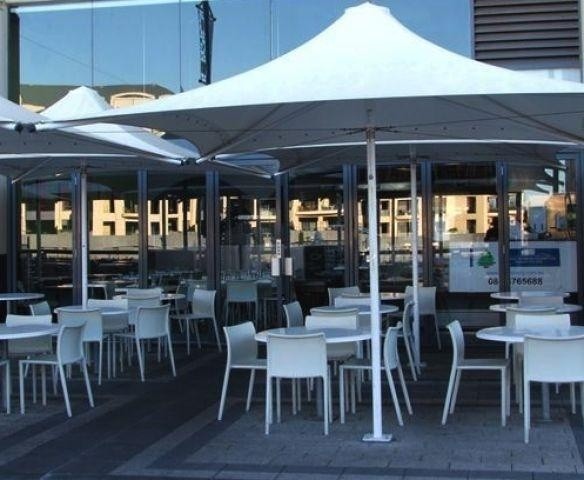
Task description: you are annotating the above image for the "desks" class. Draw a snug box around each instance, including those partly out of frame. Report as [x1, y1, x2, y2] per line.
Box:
[255, 325, 372, 422]
[476, 324, 583, 424]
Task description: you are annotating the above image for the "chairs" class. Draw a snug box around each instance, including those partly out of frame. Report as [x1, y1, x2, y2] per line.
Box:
[442, 319, 511, 427]
[0, 274, 271, 417]
[284, 284, 584, 413]
[338, 321, 413, 426]
[266, 331, 334, 435]
[523, 336, 584, 446]
[216, 322, 297, 425]
